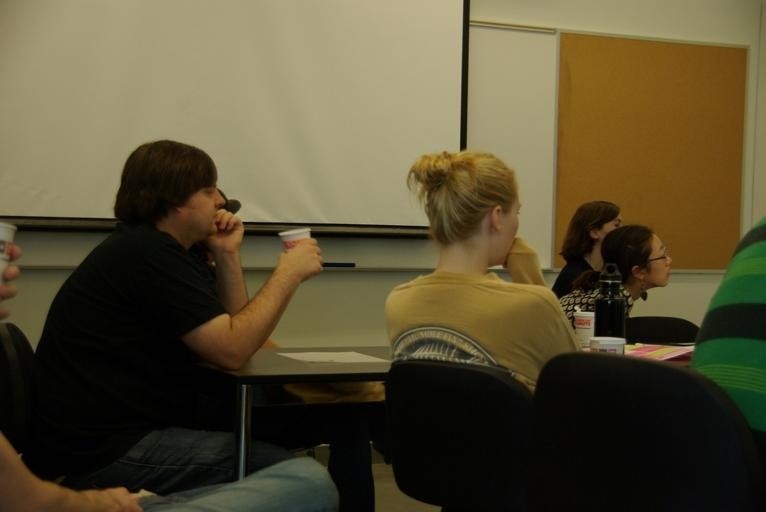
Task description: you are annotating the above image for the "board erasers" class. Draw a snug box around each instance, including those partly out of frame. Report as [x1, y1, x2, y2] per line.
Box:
[322, 263, 356, 267]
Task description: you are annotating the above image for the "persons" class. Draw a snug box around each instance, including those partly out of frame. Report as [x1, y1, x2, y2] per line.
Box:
[385, 150, 584, 398]
[558, 225, 672, 333]
[552, 200, 623, 300]
[689, 214, 766, 472]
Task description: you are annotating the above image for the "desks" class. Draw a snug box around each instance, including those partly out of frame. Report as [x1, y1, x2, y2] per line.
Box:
[225, 338, 398, 510]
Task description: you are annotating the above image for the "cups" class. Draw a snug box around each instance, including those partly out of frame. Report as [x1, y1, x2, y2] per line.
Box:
[591, 338, 626, 356]
[574, 311, 596, 344]
[279, 228, 311, 250]
[0, 221, 18, 281]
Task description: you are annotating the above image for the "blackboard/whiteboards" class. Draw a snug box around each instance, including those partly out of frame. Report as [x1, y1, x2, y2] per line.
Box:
[7, 20, 559, 273]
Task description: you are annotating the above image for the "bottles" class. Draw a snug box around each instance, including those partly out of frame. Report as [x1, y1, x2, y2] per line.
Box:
[595, 263, 625, 337]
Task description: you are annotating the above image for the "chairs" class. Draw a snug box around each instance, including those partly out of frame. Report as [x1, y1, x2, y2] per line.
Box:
[624, 316, 701, 346]
[383, 354, 534, 511]
[530, 352, 764, 510]
